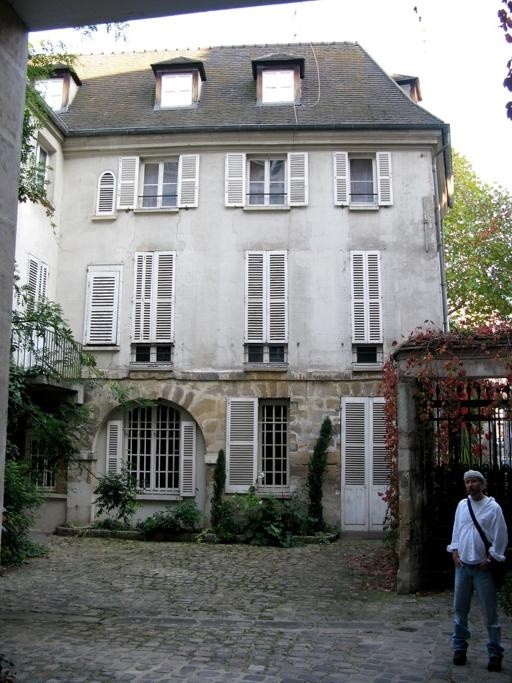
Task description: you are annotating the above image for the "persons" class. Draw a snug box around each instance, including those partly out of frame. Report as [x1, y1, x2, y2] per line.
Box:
[445, 468, 509, 673]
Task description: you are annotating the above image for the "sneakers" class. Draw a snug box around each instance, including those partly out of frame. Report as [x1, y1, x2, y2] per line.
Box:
[486, 655, 504, 672]
[452, 650, 467, 666]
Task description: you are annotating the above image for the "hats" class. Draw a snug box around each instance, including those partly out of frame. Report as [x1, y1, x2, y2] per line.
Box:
[463, 468, 485, 482]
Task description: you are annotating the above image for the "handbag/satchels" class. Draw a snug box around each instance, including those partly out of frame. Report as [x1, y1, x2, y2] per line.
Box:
[484, 541, 504, 593]
